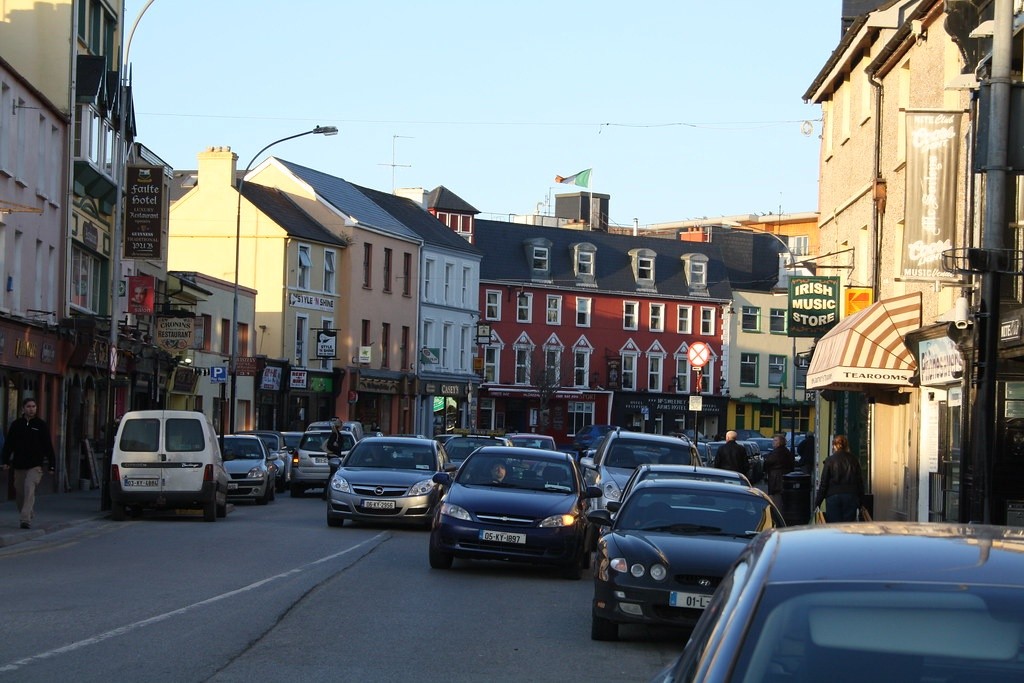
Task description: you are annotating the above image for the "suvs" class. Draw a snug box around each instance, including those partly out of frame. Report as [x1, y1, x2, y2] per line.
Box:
[288, 431, 358, 497]
[584, 427, 704, 541]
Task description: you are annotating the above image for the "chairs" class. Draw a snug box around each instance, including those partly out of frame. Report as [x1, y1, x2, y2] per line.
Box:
[518, 285, 528, 298]
[307, 441, 321, 449]
[543, 466, 568, 485]
[722, 509, 750, 533]
[613, 447, 634, 463]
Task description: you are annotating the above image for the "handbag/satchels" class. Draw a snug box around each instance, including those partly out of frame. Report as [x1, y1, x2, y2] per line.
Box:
[808, 506, 826, 525]
[861, 506, 872, 521]
[321, 438, 338, 454]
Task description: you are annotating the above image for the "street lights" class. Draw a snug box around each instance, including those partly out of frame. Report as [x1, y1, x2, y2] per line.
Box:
[722, 222, 795, 460]
[231, 127, 338, 425]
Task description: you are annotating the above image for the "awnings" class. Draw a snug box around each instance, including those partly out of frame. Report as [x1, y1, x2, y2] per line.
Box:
[805, 290, 922, 393]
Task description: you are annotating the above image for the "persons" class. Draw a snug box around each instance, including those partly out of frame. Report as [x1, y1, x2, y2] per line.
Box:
[322, 419, 343, 501]
[371, 422, 380, 432]
[764, 434, 794, 528]
[0, 398, 57, 529]
[488, 460, 507, 487]
[813, 434, 863, 523]
[364, 446, 386, 466]
[797, 431, 814, 476]
[714, 430, 750, 475]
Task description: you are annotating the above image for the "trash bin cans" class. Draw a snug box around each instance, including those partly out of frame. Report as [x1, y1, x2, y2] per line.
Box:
[780, 471, 811, 525]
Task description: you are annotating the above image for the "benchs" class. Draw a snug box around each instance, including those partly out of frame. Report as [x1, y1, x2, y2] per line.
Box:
[509, 478, 544, 488]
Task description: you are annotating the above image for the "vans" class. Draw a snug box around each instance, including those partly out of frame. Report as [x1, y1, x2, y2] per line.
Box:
[308, 422, 364, 449]
[110, 408, 232, 521]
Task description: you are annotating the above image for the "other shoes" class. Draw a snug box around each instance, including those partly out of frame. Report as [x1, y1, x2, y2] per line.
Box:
[20, 522, 31, 530]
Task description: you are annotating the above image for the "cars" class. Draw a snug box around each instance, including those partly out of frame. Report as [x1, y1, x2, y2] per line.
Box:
[216, 434, 278, 503]
[433, 432, 581, 474]
[587, 464, 785, 641]
[660, 430, 806, 484]
[326, 434, 456, 530]
[573, 425, 628, 449]
[430, 444, 603, 580]
[651, 520, 1024, 683]
[239, 430, 324, 493]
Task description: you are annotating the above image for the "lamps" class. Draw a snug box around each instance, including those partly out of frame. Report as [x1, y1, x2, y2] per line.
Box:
[621, 372, 629, 385]
[728, 308, 736, 317]
[716, 377, 726, 393]
[669, 375, 678, 391]
[590, 371, 599, 383]
[12, 315, 93, 347]
[794, 350, 812, 367]
